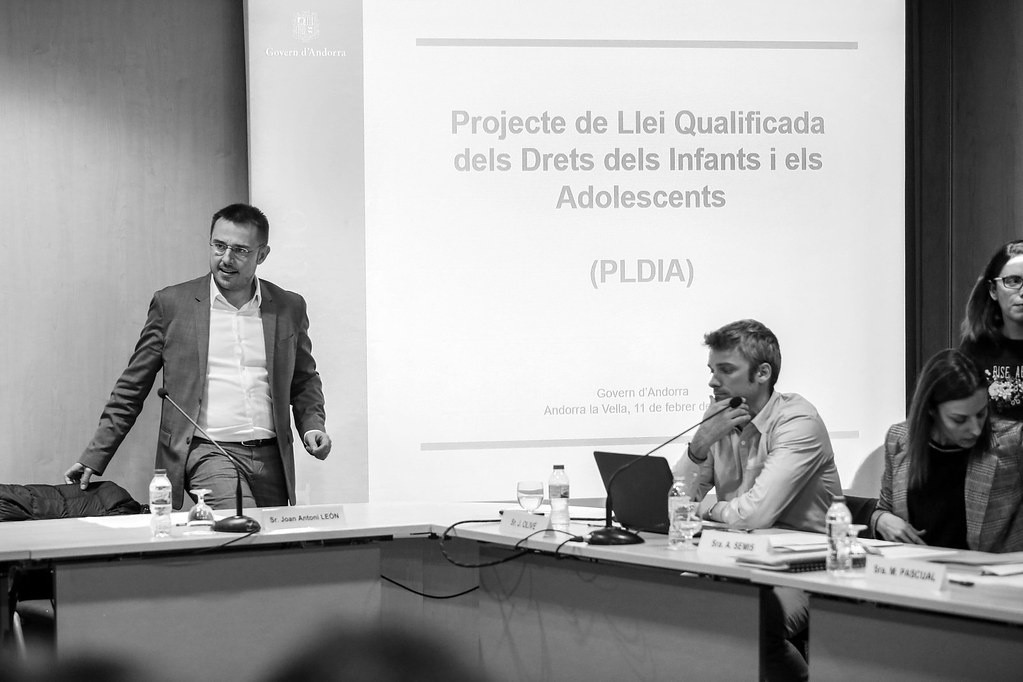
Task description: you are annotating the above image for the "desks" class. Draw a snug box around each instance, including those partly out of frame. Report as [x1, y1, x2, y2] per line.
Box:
[0, 497, 1022, 682]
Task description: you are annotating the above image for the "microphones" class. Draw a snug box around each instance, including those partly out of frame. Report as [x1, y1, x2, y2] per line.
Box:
[157, 388, 261, 533]
[587, 396, 743, 546]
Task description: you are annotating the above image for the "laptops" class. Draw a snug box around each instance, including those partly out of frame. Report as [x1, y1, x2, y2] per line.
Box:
[594, 452, 674, 534]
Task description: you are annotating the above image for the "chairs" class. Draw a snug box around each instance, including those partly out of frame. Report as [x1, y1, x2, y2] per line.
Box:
[0, 480, 152, 659]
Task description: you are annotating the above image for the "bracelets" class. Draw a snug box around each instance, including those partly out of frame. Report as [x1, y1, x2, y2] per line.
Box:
[687, 442, 707, 461]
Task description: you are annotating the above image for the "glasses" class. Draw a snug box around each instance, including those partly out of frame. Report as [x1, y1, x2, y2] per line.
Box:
[209, 241, 265, 259]
[990, 275, 1023, 290]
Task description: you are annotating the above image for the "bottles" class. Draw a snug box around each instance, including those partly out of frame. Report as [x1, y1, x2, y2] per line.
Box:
[149, 467, 173, 538]
[666, 474, 690, 549]
[548, 464, 571, 527]
[825, 495, 852, 577]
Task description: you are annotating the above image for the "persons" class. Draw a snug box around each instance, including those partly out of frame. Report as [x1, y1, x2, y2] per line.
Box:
[865, 348, 1023, 552]
[957, 241, 1023, 423]
[62, 203, 332, 512]
[674, 320, 846, 681]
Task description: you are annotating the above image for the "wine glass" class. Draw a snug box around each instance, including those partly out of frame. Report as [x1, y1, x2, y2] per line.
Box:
[516, 480, 544, 516]
[186, 488, 214, 535]
[673, 505, 702, 552]
[834, 524, 869, 577]
